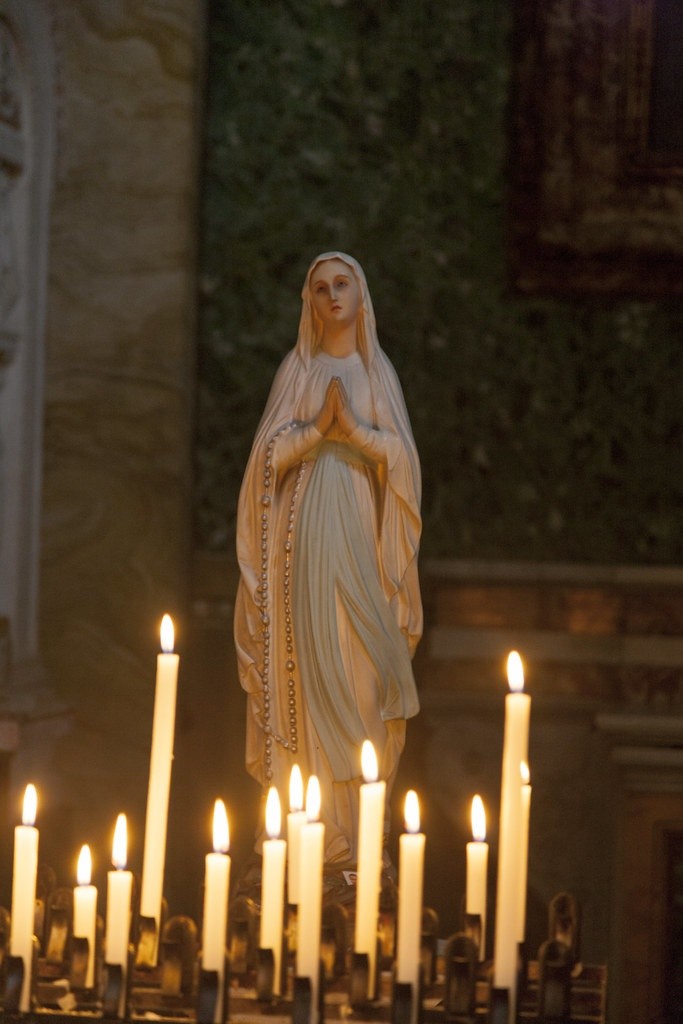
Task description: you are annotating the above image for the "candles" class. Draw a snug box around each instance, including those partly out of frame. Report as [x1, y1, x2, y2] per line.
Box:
[8, 611, 533, 1024]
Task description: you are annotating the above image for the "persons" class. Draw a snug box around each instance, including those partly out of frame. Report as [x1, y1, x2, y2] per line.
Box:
[235, 250, 423, 863]
